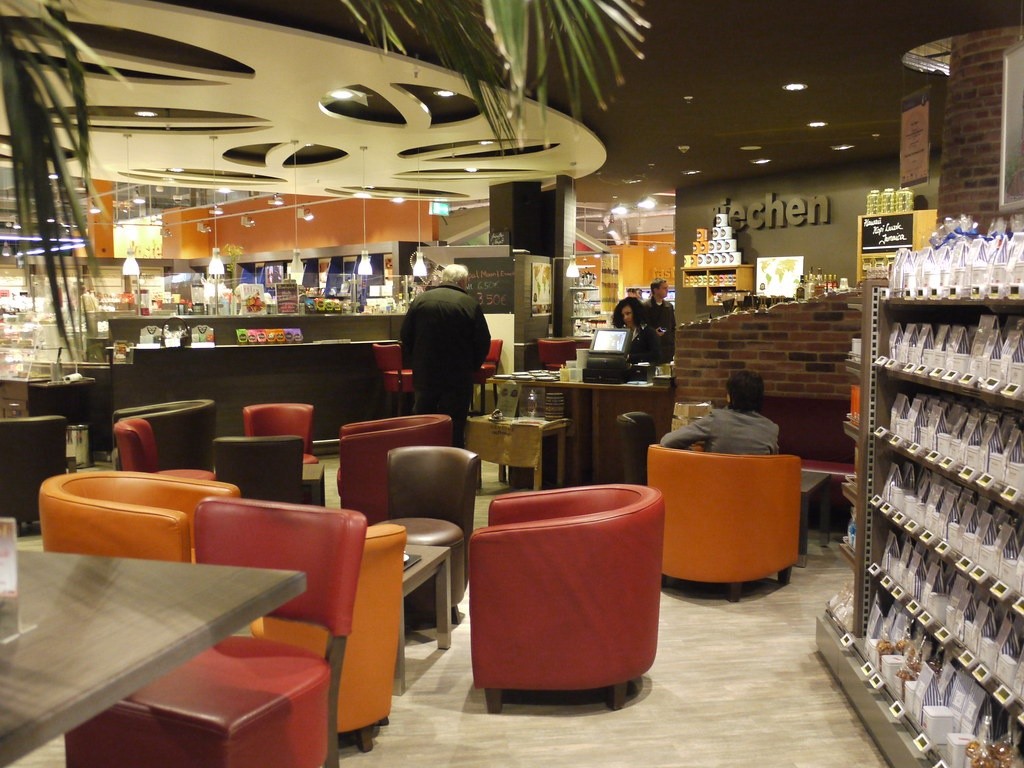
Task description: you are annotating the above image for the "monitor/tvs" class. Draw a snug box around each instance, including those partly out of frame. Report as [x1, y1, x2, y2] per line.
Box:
[587, 329, 632, 357]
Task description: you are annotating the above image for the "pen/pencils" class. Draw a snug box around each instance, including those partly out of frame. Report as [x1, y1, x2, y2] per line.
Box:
[561, 365, 563, 369]
[519, 421, 543, 424]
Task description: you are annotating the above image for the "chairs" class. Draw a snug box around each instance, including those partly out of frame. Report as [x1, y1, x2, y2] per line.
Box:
[0, 379, 802, 768]
[538, 339, 592, 368]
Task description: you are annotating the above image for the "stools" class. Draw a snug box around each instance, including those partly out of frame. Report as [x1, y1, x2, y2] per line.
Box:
[469, 339, 504, 414]
[373, 342, 414, 417]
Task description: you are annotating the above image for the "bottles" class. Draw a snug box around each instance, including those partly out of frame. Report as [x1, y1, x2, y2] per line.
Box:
[797, 268, 838, 301]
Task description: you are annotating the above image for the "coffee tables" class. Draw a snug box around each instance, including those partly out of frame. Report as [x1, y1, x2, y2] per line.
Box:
[301, 461, 325, 507]
[392, 544, 452, 697]
[795, 471, 831, 568]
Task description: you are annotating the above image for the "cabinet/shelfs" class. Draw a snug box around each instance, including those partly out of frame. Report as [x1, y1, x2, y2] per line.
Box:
[816, 273, 1024, 768]
[681, 265, 755, 305]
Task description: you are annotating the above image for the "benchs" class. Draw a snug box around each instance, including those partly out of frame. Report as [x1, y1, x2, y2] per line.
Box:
[754, 394, 855, 527]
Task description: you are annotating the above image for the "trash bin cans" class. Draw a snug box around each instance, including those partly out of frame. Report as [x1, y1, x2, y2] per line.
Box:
[65, 425, 90, 468]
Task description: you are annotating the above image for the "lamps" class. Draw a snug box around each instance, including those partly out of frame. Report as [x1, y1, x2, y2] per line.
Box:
[2, 242, 12, 258]
[111, 131, 427, 276]
[13, 217, 21, 229]
[566, 186, 676, 277]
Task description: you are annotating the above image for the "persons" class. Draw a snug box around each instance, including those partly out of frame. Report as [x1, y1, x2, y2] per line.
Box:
[627, 288, 643, 298]
[400, 264, 491, 449]
[642, 277, 676, 364]
[660, 370, 779, 454]
[610, 297, 661, 364]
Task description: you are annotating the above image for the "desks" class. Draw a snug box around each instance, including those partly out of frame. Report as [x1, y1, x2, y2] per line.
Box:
[466, 415, 570, 490]
[0, 551, 307, 768]
[486, 373, 674, 490]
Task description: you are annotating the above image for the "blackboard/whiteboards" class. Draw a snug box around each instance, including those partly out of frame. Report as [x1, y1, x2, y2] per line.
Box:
[275, 283, 299, 314]
[454, 257, 515, 314]
[858, 212, 914, 258]
